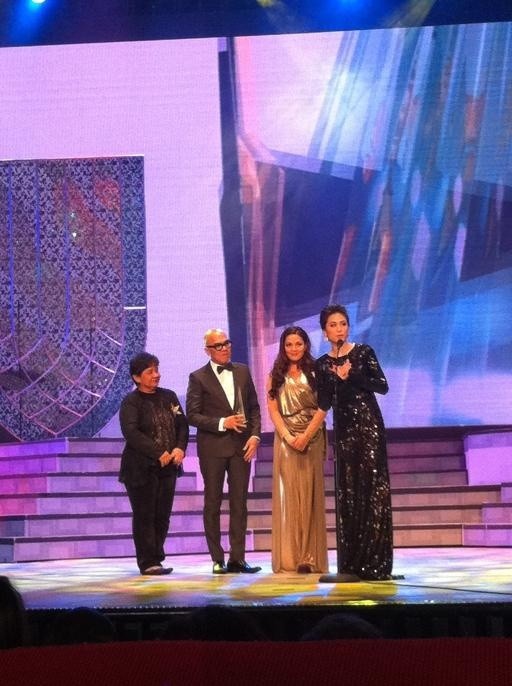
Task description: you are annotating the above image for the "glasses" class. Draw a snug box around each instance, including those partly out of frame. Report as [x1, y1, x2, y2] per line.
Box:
[206, 339, 233, 351]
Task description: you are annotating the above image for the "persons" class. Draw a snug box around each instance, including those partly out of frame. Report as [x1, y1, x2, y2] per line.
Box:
[315, 304, 405, 581]
[185, 328, 264, 575]
[117, 352, 191, 576]
[266, 325, 331, 574]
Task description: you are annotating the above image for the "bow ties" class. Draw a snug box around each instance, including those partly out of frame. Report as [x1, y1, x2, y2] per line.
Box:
[217, 362, 233, 374]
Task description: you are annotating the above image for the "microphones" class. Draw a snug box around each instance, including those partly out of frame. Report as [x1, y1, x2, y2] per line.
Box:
[337, 339, 344, 350]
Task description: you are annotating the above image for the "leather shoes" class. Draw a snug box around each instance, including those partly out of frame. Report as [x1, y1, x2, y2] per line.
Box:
[140, 563, 173, 575]
[213, 560, 226, 574]
[227, 560, 261, 573]
